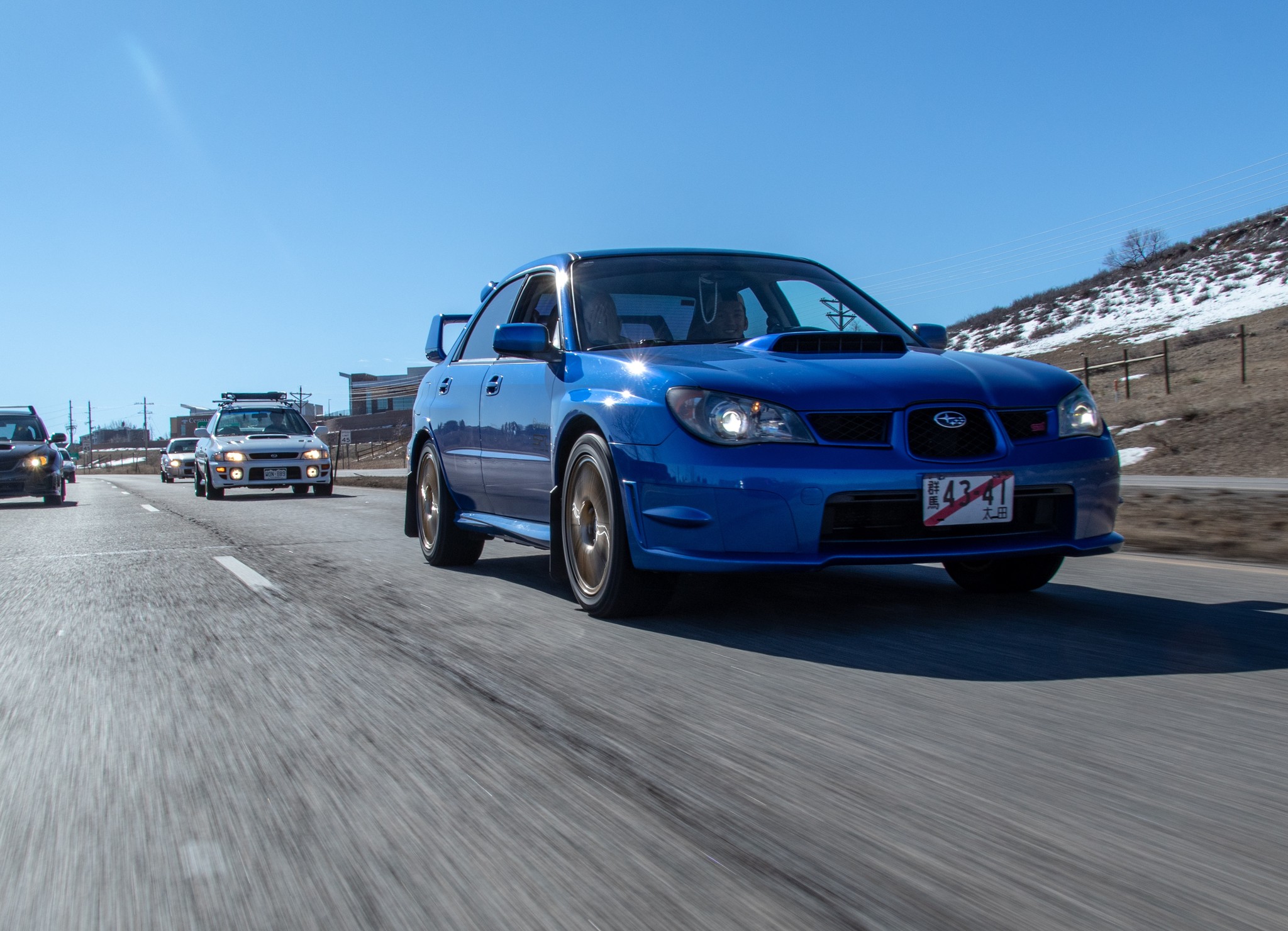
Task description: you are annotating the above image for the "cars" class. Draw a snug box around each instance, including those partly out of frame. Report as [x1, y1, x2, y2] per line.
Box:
[194, 392, 334, 500]
[160, 436, 201, 483]
[58, 447, 76, 483]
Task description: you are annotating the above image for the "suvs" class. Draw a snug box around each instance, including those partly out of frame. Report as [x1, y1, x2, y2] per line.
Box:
[402, 247, 1124, 621]
[0, 404, 67, 507]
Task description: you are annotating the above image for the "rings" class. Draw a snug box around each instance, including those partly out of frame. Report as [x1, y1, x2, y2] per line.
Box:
[599, 322, 602, 324]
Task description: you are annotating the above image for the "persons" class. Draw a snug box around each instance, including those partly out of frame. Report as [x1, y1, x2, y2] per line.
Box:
[15, 426, 35, 440]
[582, 291, 634, 348]
[263, 410, 287, 433]
[705, 290, 748, 339]
